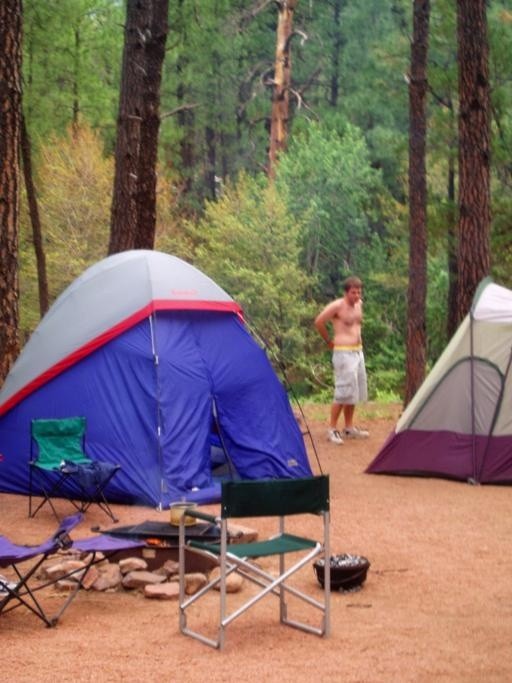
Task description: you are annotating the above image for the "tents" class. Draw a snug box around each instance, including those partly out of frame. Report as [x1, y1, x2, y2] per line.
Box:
[0, 249, 314, 510]
[364, 276, 512, 484]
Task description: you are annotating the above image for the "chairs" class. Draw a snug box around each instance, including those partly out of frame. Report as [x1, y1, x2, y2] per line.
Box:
[0, 511, 149, 630]
[177, 475, 333, 650]
[26, 414, 123, 529]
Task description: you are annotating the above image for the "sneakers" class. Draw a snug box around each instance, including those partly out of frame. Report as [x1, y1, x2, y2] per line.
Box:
[328, 427, 370, 445]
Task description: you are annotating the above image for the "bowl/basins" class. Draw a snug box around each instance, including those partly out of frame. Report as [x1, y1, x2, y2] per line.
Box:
[313, 555, 368, 588]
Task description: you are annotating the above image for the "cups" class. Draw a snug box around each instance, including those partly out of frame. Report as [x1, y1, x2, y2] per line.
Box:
[169, 502, 197, 528]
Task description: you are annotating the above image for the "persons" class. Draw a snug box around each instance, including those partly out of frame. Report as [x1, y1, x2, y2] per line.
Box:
[315, 276, 370, 445]
